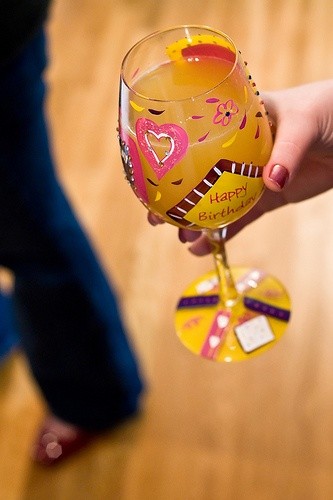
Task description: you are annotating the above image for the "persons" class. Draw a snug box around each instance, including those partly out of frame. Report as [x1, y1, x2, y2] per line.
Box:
[0, 2, 146, 469]
[148, 73, 332, 256]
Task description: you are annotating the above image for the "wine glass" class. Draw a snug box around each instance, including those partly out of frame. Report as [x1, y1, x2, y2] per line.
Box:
[116, 24, 292, 363]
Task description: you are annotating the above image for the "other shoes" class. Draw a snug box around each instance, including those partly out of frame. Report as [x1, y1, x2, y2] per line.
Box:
[32, 416, 117, 468]
